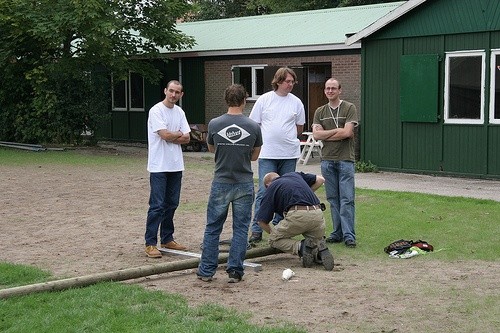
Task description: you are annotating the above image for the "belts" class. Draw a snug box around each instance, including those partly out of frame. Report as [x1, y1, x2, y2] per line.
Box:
[290, 204, 320, 210]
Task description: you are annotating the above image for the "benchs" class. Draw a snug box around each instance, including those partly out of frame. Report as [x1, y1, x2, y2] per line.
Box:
[298, 139, 321, 147]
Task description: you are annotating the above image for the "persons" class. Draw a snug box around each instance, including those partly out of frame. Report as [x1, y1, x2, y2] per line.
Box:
[256, 171, 334, 271]
[249, 67, 305, 243]
[144, 80, 191, 258]
[312, 78, 359, 246]
[196, 84, 263, 283]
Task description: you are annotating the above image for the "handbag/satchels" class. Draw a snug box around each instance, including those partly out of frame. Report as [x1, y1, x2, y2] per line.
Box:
[384, 240, 413, 256]
[410, 240, 433, 253]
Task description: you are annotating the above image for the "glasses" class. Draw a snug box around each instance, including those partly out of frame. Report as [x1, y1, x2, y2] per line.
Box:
[284, 80, 295, 84]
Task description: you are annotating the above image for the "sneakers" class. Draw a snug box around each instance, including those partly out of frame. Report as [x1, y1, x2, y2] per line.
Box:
[161, 240, 187, 250]
[145, 245, 162, 257]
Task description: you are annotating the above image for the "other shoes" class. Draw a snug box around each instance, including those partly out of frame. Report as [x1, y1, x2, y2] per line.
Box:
[248, 232, 262, 242]
[317, 242, 334, 271]
[196, 274, 213, 281]
[346, 240, 356, 248]
[228, 272, 241, 282]
[299, 239, 314, 267]
[326, 236, 337, 242]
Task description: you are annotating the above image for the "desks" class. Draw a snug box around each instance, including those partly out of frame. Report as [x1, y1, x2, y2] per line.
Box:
[297, 130, 323, 166]
